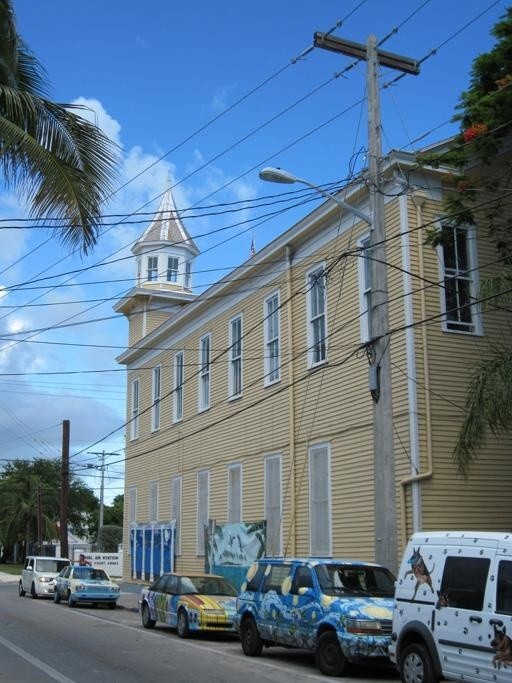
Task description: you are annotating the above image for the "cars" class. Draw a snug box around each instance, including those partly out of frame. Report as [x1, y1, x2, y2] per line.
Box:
[137, 571, 241, 636]
[53, 564, 121, 609]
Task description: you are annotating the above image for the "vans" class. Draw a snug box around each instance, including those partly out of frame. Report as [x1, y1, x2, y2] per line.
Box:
[232, 555, 398, 676]
[387, 529, 512, 681]
[17, 554, 73, 599]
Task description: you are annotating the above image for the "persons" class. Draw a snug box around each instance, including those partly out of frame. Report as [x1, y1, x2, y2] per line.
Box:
[70, 552, 93, 567]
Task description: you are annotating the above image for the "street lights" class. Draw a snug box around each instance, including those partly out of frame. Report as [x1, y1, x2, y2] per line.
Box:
[259, 165, 422, 570]
